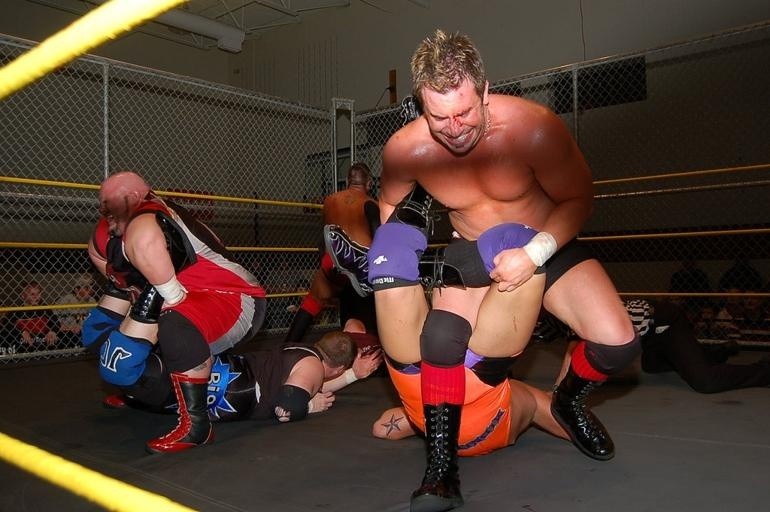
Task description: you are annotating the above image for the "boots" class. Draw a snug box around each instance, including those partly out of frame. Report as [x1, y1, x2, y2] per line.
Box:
[393, 93, 435, 230]
[128, 212, 198, 324]
[143, 372, 216, 455]
[409, 403, 463, 512]
[102, 390, 125, 408]
[324, 223, 464, 298]
[550, 359, 616, 460]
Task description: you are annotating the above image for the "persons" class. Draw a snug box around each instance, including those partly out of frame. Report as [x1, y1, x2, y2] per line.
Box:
[380, 28, 640, 512]
[323, 183, 574, 457]
[101, 173, 267, 454]
[252, 265, 310, 329]
[720, 252, 761, 289]
[83, 210, 383, 423]
[626, 299, 770, 393]
[658, 288, 770, 343]
[1, 278, 97, 356]
[286, 166, 380, 344]
